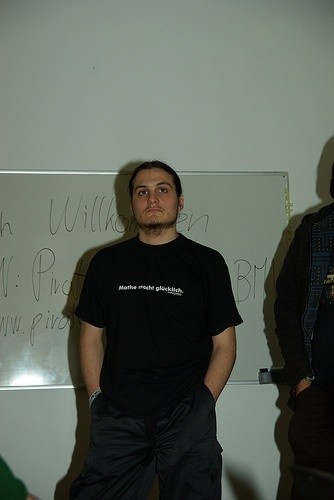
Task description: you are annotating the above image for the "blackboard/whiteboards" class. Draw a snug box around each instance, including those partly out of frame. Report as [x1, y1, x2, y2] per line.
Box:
[1, 167, 293, 394]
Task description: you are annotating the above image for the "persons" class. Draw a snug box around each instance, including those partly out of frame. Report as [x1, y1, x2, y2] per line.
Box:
[274, 163, 334, 500]
[66, 161, 244, 500]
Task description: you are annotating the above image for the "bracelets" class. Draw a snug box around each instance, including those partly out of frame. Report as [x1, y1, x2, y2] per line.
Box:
[89, 389, 102, 408]
